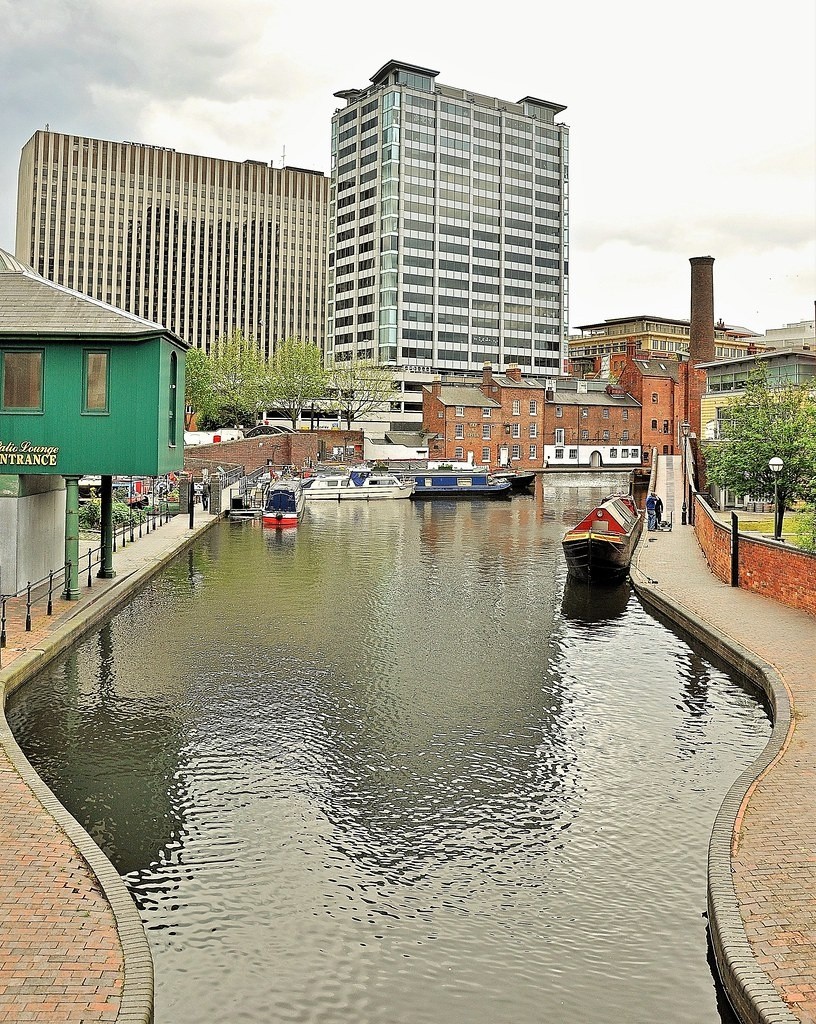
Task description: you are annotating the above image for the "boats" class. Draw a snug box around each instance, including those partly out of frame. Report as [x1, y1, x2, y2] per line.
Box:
[562, 491, 643, 585]
[262, 478, 306, 525]
[293, 466, 537, 500]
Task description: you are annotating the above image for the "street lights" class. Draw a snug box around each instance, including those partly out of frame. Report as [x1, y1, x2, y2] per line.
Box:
[768, 455, 784, 538]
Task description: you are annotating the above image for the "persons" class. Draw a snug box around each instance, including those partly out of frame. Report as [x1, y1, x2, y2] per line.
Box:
[487, 474, 493, 482]
[201, 479, 211, 511]
[654, 493, 663, 529]
[646, 492, 659, 531]
[507, 457, 512, 468]
